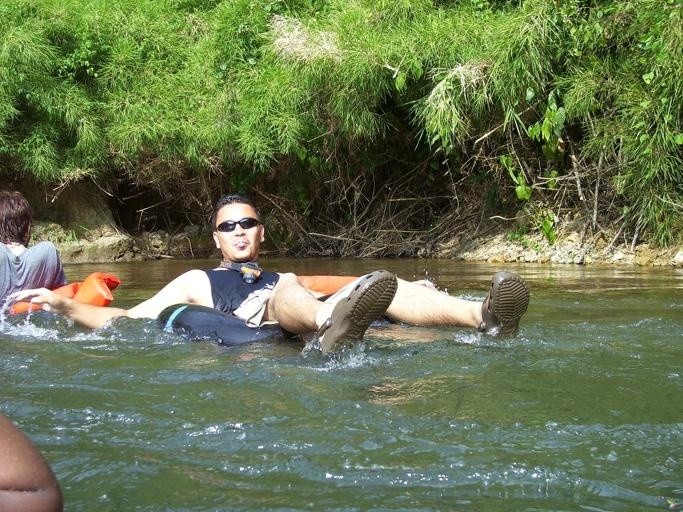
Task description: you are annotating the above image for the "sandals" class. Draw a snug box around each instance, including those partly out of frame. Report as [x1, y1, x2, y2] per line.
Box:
[313, 269, 399, 357]
[479, 270, 528, 341]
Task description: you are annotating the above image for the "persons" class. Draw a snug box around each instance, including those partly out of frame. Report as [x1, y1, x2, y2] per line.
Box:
[7, 194, 529, 357]
[0, 188, 66, 313]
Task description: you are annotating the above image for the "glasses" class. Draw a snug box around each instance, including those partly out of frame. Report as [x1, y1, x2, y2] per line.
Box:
[213, 217, 262, 233]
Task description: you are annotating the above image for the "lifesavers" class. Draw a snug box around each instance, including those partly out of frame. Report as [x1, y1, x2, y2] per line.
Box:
[157, 297, 396, 347]
